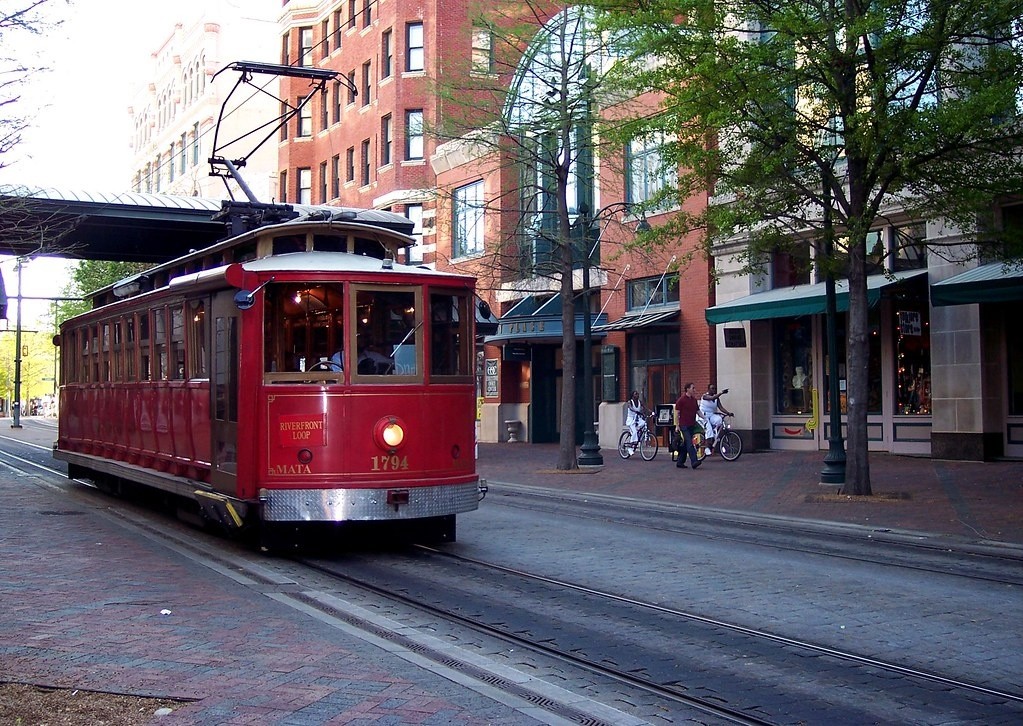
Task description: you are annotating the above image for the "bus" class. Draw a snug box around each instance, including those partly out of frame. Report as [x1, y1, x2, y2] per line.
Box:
[50, 57, 497, 553]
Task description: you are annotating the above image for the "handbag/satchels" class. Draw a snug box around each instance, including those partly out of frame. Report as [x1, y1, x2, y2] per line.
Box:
[672, 430, 683, 451]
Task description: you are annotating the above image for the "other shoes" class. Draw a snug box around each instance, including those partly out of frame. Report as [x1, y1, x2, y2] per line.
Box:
[722, 446, 726, 453]
[628, 448, 635, 456]
[676, 465, 688, 468]
[704, 447, 712, 456]
[692, 461, 702, 469]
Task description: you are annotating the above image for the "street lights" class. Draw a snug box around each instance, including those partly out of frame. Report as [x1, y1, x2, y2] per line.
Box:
[576, 199, 653, 464]
[817, 131, 897, 487]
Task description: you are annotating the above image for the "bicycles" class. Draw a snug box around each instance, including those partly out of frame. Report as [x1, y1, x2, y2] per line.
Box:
[689, 411, 743, 462]
[617, 412, 659, 461]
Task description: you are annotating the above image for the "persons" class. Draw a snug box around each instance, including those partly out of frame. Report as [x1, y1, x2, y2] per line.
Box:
[40, 393, 58, 419]
[625, 390, 655, 455]
[671, 382, 708, 469]
[271, 350, 305, 385]
[11, 401, 15, 418]
[330, 324, 395, 375]
[696, 383, 735, 455]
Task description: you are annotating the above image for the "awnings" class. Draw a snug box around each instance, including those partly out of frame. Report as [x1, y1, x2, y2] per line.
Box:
[930, 257, 1023, 307]
[591, 309, 680, 332]
[705, 269, 927, 326]
